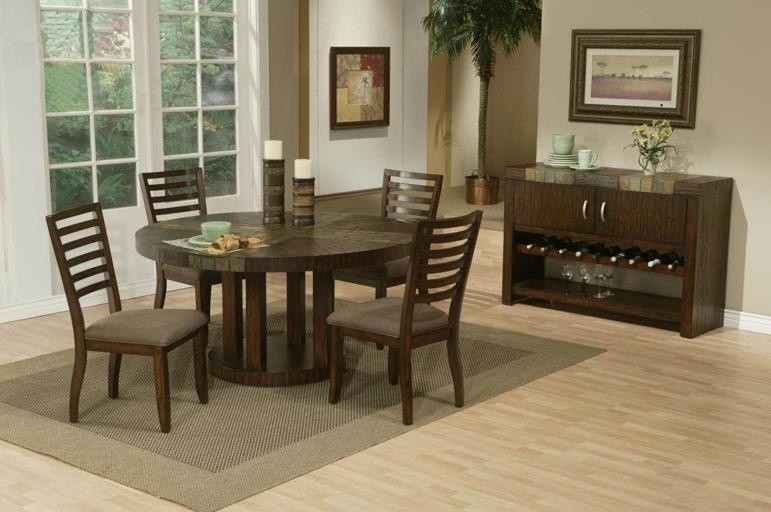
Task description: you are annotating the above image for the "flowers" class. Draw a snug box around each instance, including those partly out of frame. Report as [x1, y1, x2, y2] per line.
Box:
[627, 118, 675, 160]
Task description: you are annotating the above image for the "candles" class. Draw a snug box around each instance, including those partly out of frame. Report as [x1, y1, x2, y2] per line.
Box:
[293, 158, 310, 179]
[263, 139, 283, 160]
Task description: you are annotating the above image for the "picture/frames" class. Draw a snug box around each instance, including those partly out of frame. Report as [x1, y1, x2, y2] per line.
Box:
[329, 43, 391, 131]
[569, 27, 701, 130]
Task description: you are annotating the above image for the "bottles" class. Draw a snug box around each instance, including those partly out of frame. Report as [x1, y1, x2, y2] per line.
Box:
[521, 234, 683, 272]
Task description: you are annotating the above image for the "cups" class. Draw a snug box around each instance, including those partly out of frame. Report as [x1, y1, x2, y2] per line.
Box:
[577, 151, 598, 167]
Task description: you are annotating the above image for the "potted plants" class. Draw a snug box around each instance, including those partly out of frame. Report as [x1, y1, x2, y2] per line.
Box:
[426, 0, 543, 205]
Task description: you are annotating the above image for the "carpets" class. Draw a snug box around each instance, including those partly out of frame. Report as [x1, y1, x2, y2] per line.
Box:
[0, 293, 606, 512]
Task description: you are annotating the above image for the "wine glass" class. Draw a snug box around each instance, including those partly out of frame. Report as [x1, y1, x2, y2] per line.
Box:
[561, 259, 618, 302]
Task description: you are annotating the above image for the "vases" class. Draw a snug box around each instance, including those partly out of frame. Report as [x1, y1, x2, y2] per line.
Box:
[641, 157, 658, 177]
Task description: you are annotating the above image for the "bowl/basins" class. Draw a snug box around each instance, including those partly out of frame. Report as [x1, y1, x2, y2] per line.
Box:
[202, 220, 232, 242]
[552, 132, 575, 155]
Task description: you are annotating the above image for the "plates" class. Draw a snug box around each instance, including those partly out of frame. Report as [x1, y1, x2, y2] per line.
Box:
[188, 234, 238, 247]
[544, 154, 577, 168]
[567, 165, 600, 171]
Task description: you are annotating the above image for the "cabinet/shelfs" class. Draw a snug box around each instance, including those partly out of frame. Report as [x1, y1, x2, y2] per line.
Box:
[495, 163, 733, 339]
